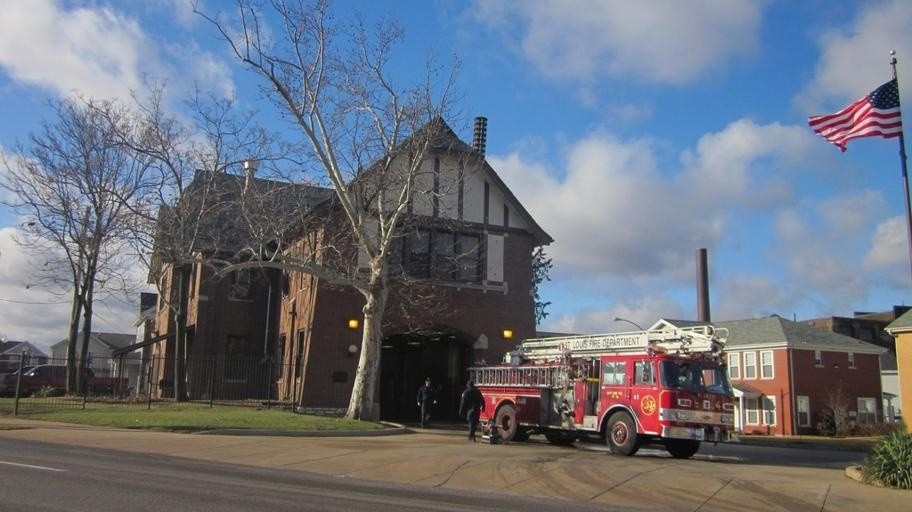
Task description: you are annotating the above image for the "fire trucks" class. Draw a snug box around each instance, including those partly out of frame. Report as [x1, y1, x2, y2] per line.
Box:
[463, 323, 736, 461]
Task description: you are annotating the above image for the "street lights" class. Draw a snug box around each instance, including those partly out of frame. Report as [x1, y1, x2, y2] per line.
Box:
[613, 317, 644, 331]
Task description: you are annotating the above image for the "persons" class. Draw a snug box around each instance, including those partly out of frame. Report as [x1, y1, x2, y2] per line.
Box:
[458, 379, 485, 442]
[416, 376, 438, 429]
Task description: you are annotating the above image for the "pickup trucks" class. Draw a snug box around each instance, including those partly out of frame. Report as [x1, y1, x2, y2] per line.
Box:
[1, 363, 130, 395]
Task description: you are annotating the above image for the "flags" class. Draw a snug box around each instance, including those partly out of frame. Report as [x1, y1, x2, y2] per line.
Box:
[808, 77, 904, 152]
